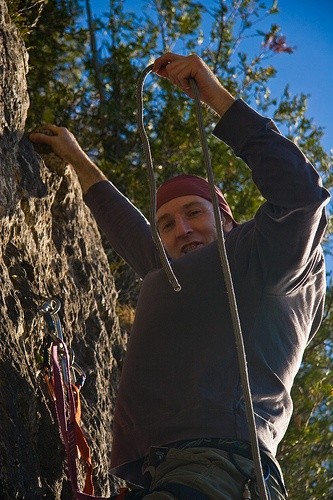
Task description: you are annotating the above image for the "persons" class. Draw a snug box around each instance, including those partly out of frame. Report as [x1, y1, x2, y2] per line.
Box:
[29, 51, 331, 499]
